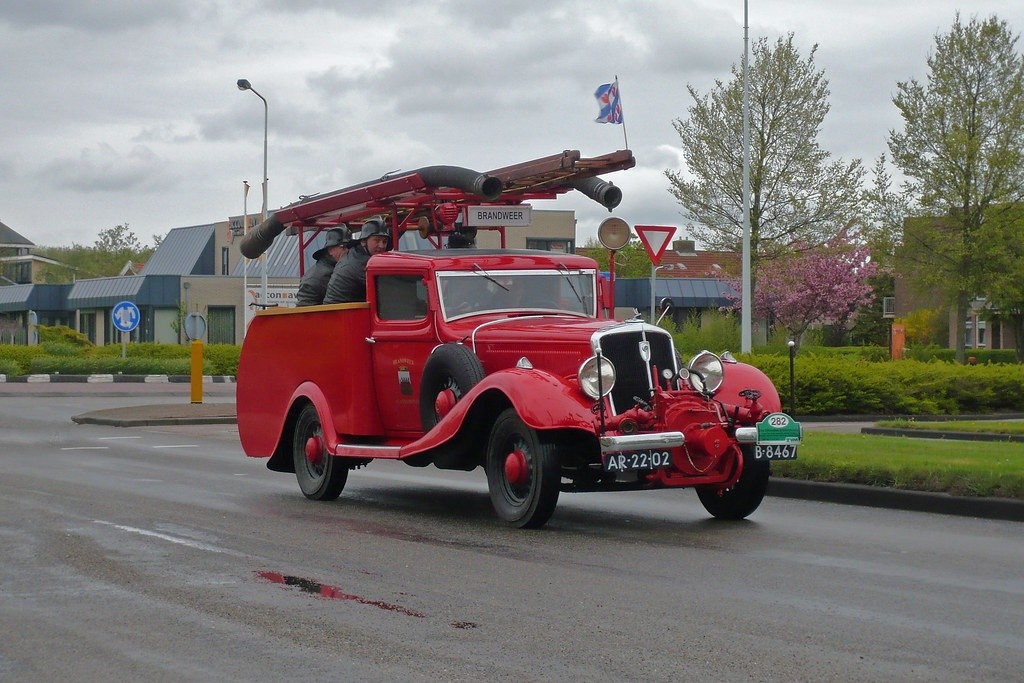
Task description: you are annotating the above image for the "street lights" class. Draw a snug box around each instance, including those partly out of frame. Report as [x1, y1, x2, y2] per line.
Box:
[237, 78, 269, 310]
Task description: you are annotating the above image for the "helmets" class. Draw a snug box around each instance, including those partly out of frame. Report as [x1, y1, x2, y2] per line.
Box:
[357, 218, 392, 240]
[312, 228, 360, 260]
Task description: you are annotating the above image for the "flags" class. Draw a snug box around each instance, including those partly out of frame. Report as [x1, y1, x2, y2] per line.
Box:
[593, 79, 623, 125]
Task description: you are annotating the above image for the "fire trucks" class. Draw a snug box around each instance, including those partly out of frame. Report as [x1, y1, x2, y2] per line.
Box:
[234, 75, 803, 531]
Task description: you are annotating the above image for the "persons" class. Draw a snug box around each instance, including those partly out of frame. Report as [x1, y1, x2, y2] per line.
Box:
[320, 219, 394, 307]
[293, 227, 353, 308]
[500, 277, 523, 307]
